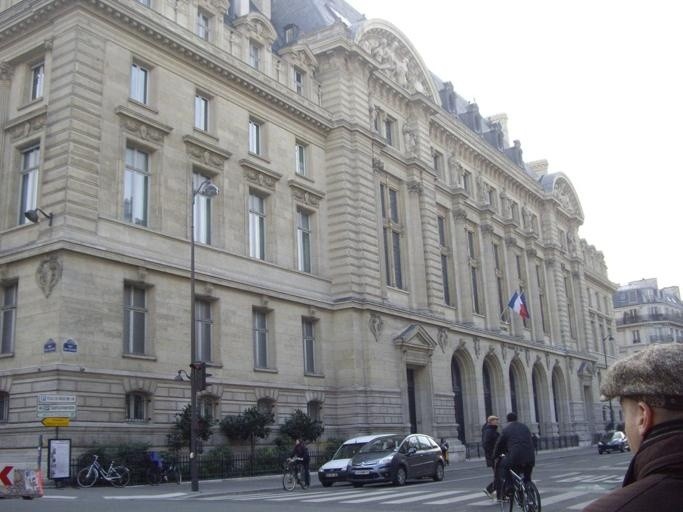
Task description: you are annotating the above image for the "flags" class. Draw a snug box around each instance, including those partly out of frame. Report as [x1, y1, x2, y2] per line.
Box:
[507, 291, 529, 320]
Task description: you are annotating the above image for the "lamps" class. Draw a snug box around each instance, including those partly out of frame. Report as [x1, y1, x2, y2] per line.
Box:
[23, 207, 55, 226]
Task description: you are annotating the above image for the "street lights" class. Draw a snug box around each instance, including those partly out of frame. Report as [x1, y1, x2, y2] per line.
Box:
[189, 178, 220, 492]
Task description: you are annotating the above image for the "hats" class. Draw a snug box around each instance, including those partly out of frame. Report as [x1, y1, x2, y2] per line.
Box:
[488, 416, 500, 423]
[599, 342, 683, 410]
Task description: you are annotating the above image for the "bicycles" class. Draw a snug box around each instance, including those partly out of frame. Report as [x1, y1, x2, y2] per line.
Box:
[282, 458, 312, 492]
[494, 454, 541, 512]
[145, 452, 183, 486]
[76, 454, 131, 489]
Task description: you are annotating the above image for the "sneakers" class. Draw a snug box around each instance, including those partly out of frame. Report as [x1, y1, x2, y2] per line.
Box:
[483, 489, 493, 499]
[496, 499, 510, 502]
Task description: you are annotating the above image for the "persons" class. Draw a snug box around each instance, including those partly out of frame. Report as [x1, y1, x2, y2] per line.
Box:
[482, 413, 538, 500]
[580, 342, 683, 512]
[439, 438, 449, 468]
[286, 439, 311, 489]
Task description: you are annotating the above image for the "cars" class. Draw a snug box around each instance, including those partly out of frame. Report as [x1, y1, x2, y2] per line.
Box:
[597, 430, 631, 455]
[317, 433, 398, 488]
[346, 433, 445, 488]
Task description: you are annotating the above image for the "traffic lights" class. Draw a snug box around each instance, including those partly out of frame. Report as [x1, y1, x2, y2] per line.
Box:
[197, 361, 213, 392]
